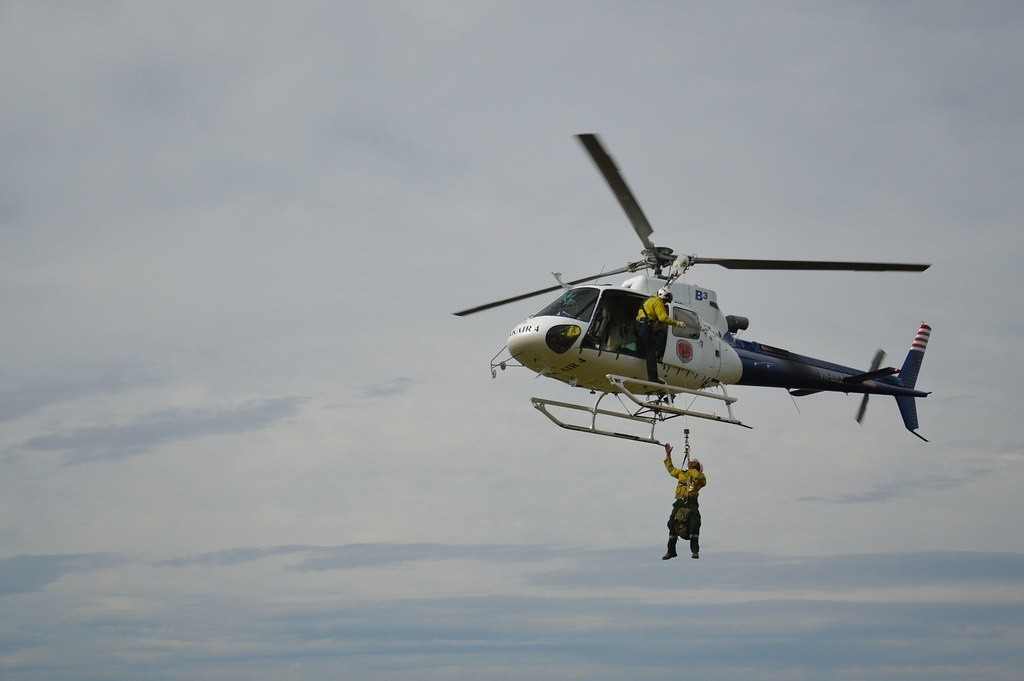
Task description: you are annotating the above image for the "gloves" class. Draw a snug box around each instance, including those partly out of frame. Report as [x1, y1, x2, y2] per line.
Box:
[676, 321, 686, 328]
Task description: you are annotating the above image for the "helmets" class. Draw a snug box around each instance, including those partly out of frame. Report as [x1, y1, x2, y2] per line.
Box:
[658, 287, 673, 303]
[699, 463, 703, 473]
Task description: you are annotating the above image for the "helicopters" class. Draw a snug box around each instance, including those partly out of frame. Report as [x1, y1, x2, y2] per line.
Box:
[450, 131, 935, 446]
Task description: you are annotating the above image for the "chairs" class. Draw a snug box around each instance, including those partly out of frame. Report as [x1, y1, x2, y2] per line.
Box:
[588, 307, 611, 344]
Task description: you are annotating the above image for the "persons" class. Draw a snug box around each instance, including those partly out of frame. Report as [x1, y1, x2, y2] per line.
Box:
[662, 443, 707, 560]
[560, 313, 582, 338]
[633, 286, 685, 384]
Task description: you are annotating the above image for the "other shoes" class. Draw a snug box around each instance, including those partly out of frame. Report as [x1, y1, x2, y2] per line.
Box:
[691, 551, 699, 558]
[662, 551, 677, 560]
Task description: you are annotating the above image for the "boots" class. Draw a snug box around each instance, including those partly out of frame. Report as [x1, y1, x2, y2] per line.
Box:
[647, 351, 665, 384]
